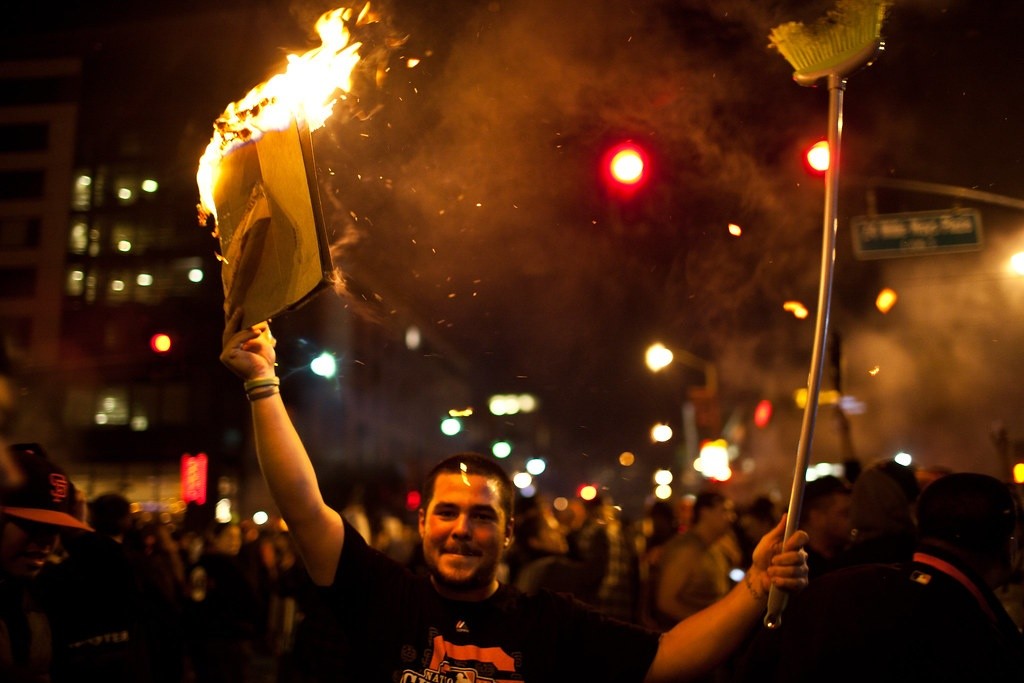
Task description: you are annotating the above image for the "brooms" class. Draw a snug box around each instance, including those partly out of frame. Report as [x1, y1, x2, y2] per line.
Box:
[766, 0, 892, 635]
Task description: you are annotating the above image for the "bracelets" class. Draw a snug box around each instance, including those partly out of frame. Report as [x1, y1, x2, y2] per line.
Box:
[243, 375, 281, 391]
[247, 386, 280, 400]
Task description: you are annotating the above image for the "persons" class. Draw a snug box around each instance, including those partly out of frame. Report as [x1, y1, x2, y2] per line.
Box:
[503, 457, 869, 683]
[36, 476, 424, 682]
[1, 442, 96, 683]
[221, 295, 811, 682]
[730, 472, 1024, 682]
[822, 457, 924, 578]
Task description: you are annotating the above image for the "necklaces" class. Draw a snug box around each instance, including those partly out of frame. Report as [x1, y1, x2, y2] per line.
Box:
[912, 550, 999, 625]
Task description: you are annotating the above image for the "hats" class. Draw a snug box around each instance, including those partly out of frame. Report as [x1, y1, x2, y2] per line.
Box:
[0, 457, 95, 532]
[86, 493, 131, 520]
